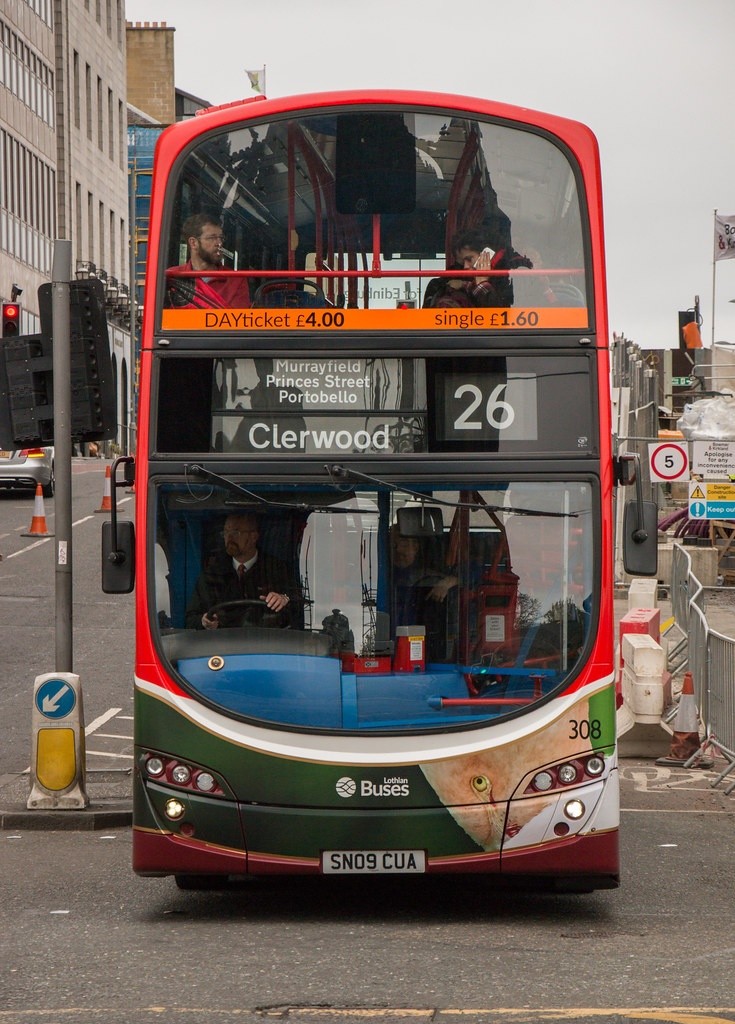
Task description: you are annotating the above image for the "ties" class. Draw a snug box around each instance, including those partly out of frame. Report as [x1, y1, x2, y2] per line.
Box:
[238, 564, 246, 581]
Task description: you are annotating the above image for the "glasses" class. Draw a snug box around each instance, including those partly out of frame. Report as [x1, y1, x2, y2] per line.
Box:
[391, 538, 418, 549]
[197, 234, 225, 242]
[221, 529, 253, 538]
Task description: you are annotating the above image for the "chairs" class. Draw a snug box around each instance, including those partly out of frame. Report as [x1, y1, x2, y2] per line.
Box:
[255, 279, 324, 309]
[201, 515, 300, 628]
[401, 573, 457, 656]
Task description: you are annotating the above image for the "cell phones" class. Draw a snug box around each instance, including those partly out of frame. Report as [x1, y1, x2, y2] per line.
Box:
[473, 247, 495, 270]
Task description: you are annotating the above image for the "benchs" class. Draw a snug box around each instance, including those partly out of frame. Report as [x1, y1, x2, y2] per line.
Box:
[385, 531, 508, 598]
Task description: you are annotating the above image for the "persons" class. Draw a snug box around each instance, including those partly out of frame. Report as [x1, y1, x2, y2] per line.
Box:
[369, 525, 461, 626]
[423, 231, 533, 306]
[164, 214, 250, 310]
[185, 513, 296, 630]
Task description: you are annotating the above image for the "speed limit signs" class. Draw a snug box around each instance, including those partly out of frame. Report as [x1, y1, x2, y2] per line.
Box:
[650, 444, 690, 481]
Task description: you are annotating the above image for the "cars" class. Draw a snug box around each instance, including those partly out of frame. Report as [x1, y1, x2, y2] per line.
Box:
[0, 445, 55, 498]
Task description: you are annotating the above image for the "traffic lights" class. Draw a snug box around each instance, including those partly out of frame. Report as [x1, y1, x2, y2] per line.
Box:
[0, 301, 23, 341]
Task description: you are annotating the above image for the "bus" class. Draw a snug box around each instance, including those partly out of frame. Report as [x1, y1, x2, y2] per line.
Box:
[102, 82, 668, 895]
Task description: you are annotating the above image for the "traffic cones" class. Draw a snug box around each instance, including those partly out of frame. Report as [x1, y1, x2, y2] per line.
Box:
[19, 483, 56, 538]
[123, 448, 135, 495]
[94, 464, 126, 513]
